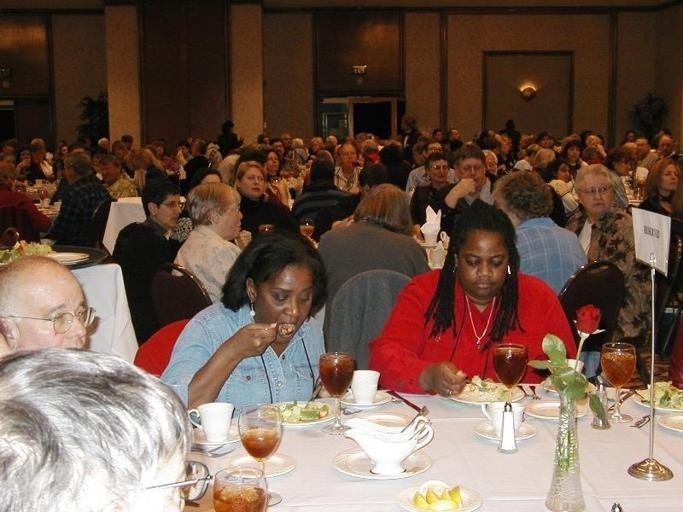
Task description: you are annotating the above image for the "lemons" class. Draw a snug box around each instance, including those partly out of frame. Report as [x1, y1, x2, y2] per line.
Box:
[412, 485, 462, 512]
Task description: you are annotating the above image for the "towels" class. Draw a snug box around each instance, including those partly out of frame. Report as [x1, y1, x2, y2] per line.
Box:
[420, 205, 442, 243]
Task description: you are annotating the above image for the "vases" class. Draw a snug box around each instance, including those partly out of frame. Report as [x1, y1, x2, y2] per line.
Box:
[545, 393, 586, 512]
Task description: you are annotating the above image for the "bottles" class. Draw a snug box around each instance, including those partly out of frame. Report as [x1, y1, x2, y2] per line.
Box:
[497, 400, 517, 452]
[592, 382, 611, 428]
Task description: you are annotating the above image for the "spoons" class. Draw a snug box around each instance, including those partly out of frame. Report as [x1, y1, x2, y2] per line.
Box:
[606, 386, 625, 403]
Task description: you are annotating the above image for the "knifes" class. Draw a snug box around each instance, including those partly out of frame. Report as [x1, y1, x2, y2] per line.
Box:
[609, 387, 635, 412]
[386, 390, 423, 413]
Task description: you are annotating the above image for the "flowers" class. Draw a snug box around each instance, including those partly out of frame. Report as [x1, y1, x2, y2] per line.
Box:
[530, 302, 606, 424]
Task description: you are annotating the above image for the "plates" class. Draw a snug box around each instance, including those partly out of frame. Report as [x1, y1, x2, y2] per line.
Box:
[451, 384, 525, 405]
[259, 400, 336, 428]
[540, 379, 597, 394]
[521, 401, 590, 420]
[396, 482, 483, 511]
[330, 446, 433, 481]
[632, 394, 683, 411]
[475, 422, 537, 441]
[220, 452, 295, 478]
[42, 252, 90, 267]
[657, 413, 683, 431]
[193, 421, 242, 446]
[338, 389, 391, 411]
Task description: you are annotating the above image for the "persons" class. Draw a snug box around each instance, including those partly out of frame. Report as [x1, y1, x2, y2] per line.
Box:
[0, 115, 683, 428]
[0, 345, 214, 512]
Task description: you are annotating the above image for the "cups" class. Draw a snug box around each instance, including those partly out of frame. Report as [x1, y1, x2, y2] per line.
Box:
[42, 199, 51, 208]
[546, 359, 584, 375]
[55, 202, 60, 211]
[35, 179, 42, 187]
[342, 415, 434, 475]
[299, 216, 314, 237]
[212, 466, 267, 511]
[258, 224, 273, 233]
[187, 401, 234, 442]
[347, 368, 381, 403]
[480, 400, 525, 435]
[270, 176, 281, 190]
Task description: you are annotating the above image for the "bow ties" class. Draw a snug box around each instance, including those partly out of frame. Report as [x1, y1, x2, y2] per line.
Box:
[401, 132, 412, 138]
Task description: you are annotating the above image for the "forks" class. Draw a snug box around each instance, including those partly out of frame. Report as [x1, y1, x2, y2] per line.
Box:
[188, 441, 235, 457]
[530, 385, 542, 400]
[516, 382, 532, 399]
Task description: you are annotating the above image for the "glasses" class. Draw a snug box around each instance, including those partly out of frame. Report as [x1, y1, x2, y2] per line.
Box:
[13, 306, 96, 334]
[581, 184, 609, 195]
[148, 461, 213, 503]
[159, 201, 185, 210]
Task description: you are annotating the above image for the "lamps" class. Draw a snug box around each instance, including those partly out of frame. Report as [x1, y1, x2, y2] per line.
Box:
[520, 87, 536, 101]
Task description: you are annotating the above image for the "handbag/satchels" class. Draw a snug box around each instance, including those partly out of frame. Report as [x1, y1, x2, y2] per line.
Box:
[656, 295, 681, 359]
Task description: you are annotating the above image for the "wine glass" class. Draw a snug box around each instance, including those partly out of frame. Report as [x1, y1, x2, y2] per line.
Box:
[599, 342, 637, 424]
[316, 353, 355, 436]
[36, 187, 47, 208]
[237, 404, 284, 506]
[636, 176, 645, 200]
[492, 343, 528, 398]
